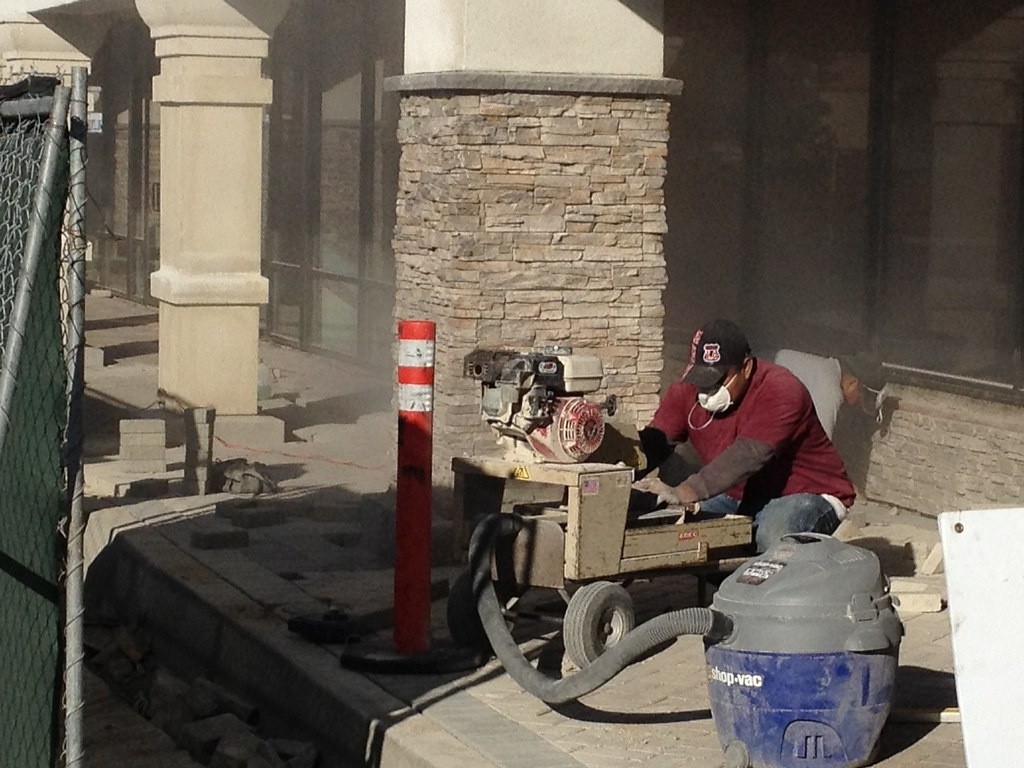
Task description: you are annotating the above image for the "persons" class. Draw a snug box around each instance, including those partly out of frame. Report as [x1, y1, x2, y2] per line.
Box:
[751, 340, 886, 441]
[627, 318, 856, 555]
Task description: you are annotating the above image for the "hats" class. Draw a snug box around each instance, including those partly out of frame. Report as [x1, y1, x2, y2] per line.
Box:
[845, 347, 884, 418]
[683, 318, 748, 387]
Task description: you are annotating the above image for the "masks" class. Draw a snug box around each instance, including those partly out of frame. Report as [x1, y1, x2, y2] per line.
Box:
[861, 382, 887, 411]
[698, 370, 748, 413]
